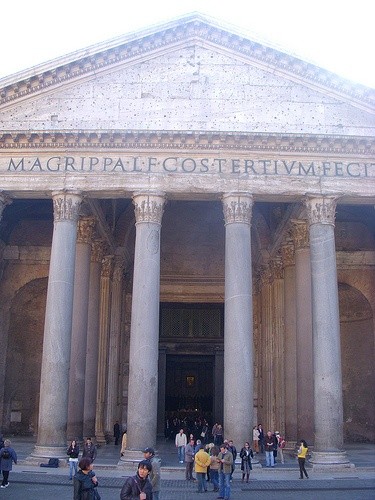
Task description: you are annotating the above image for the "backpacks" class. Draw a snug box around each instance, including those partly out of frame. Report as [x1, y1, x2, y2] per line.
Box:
[2, 449, 10, 459]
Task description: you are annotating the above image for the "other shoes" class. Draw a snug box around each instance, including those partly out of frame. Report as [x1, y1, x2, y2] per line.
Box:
[5, 482, 9, 487]
[1, 485, 6, 489]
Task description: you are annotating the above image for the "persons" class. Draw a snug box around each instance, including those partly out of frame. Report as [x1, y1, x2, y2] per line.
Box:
[240, 442, 254, 482]
[298, 440, 309, 479]
[216, 445, 233, 500]
[171, 416, 223, 463]
[120, 460, 153, 500]
[224, 440, 237, 482]
[254, 425, 264, 453]
[143, 448, 160, 500]
[74, 458, 98, 500]
[0, 435, 17, 488]
[120, 431, 127, 457]
[263, 430, 284, 467]
[67, 440, 79, 480]
[82, 438, 98, 462]
[114, 421, 120, 445]
[185, 440, 220, 492]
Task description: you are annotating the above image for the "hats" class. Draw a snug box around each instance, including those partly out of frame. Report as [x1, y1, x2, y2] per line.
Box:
[143, 448, 154, 453]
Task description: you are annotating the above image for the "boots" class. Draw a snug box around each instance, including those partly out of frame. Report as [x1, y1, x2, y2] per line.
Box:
[242, 473, 245, 483]
[246, 473, 250, 483]
